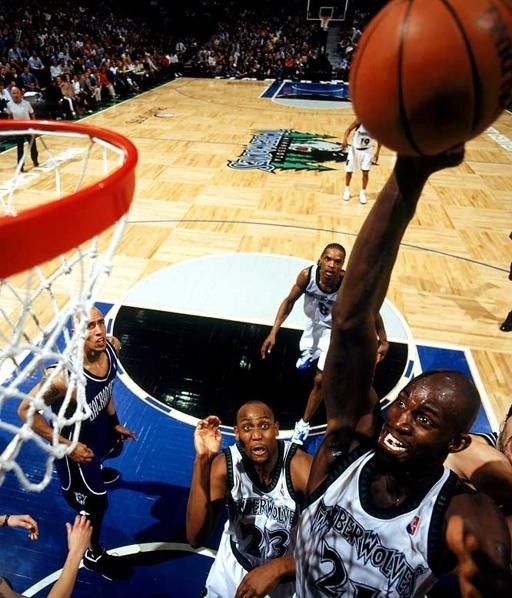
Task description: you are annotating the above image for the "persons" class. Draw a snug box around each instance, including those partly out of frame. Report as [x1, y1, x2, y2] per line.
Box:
[442, 405, 512, 519]
[184, 397, 315, 596]
[18, 306, 138, 583]
[260, 241, 390, 449]
[0, 505, 94, 598]
[291, 123, 511, 596]
[1, 2, 388, 130]
[6, 85, 43, 176]
[341, 117, 384, 204]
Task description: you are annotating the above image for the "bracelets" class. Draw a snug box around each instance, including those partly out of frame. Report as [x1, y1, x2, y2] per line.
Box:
[109, 411, 121, 427]
[2, 513, 12, 529]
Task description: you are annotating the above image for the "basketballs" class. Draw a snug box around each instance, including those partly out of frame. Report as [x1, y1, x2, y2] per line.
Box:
[350, 1, 512, 158]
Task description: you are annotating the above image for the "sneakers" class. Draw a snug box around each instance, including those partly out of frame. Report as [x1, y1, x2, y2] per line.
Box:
[34, 161, 39, 167]
[359, 192, 367, 204]
[84, 547, 127, 582]
[342, 190, 350, 201]
[291, 420, 310, 447]
[296, 348, 322, 370]
